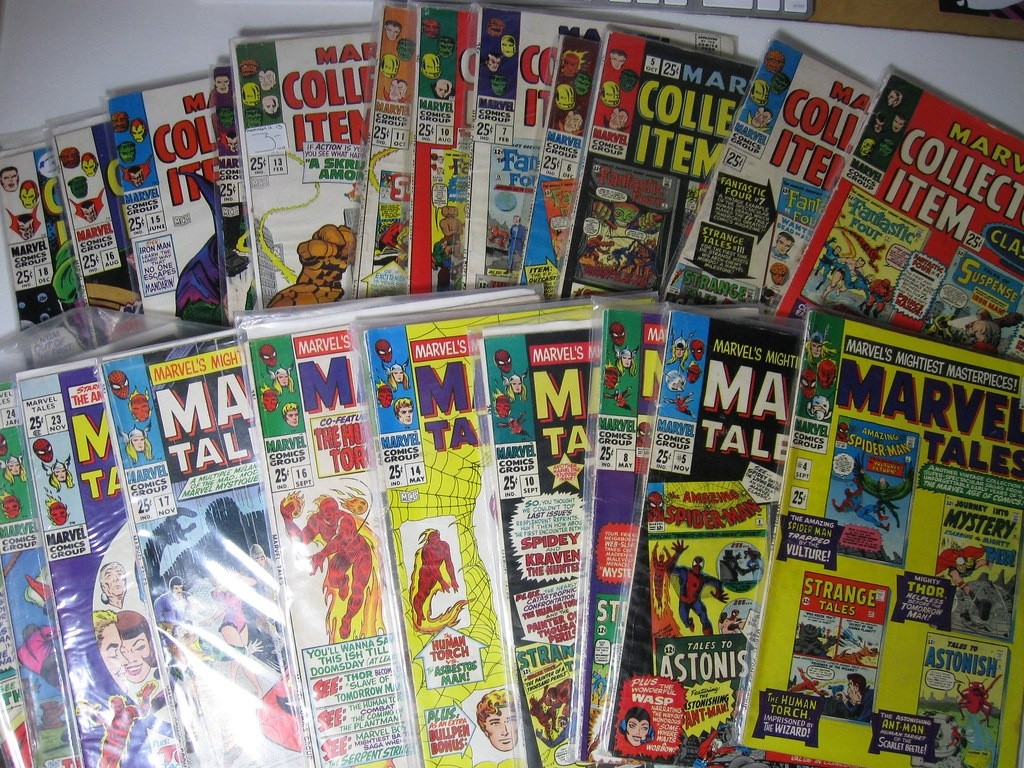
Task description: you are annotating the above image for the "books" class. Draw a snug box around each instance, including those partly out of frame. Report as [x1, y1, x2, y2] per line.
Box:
[0, 1, 1023, 768]
